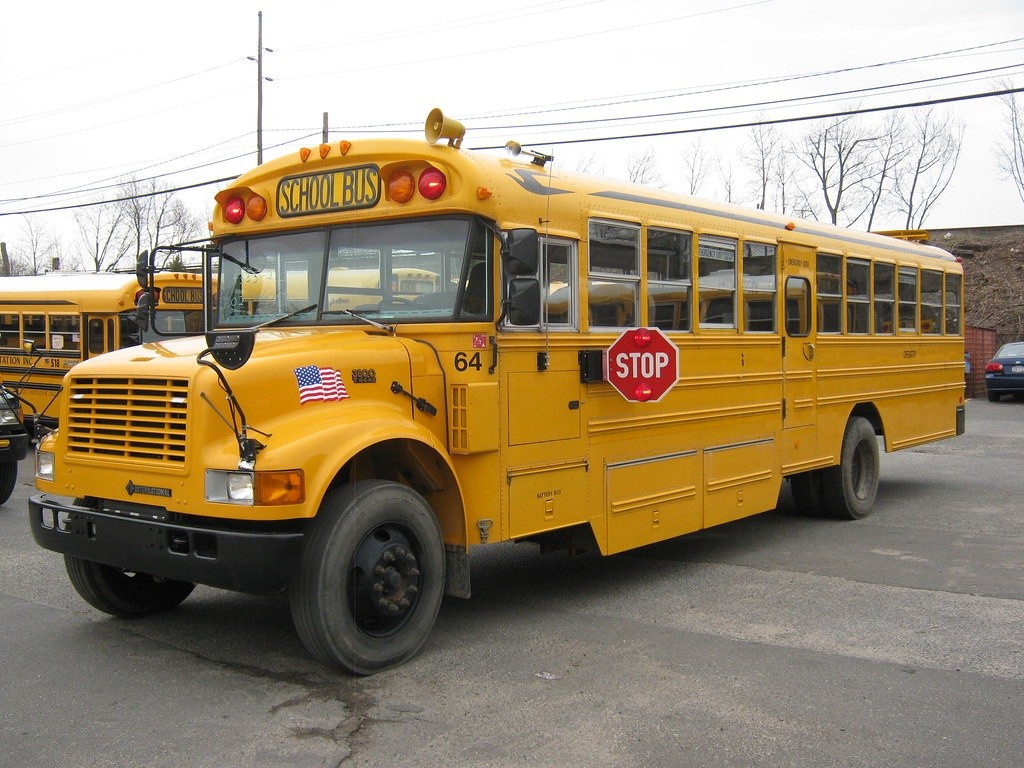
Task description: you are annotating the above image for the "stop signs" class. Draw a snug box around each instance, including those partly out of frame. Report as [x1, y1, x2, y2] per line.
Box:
[606, 326, 679, 404]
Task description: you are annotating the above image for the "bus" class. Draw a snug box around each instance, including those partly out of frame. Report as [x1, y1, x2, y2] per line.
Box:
[27, 108, 972, 674]
[545, 264, 856, 337]
[0, 271, 253, 444]
[0, 338, 63, 506]
[255, 268, 450, 315]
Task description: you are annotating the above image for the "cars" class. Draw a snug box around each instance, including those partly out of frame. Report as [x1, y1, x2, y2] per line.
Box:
[983, 341, 1024, 402]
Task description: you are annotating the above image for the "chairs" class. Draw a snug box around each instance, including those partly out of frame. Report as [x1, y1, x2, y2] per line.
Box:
[462, 262, 486, 316]
